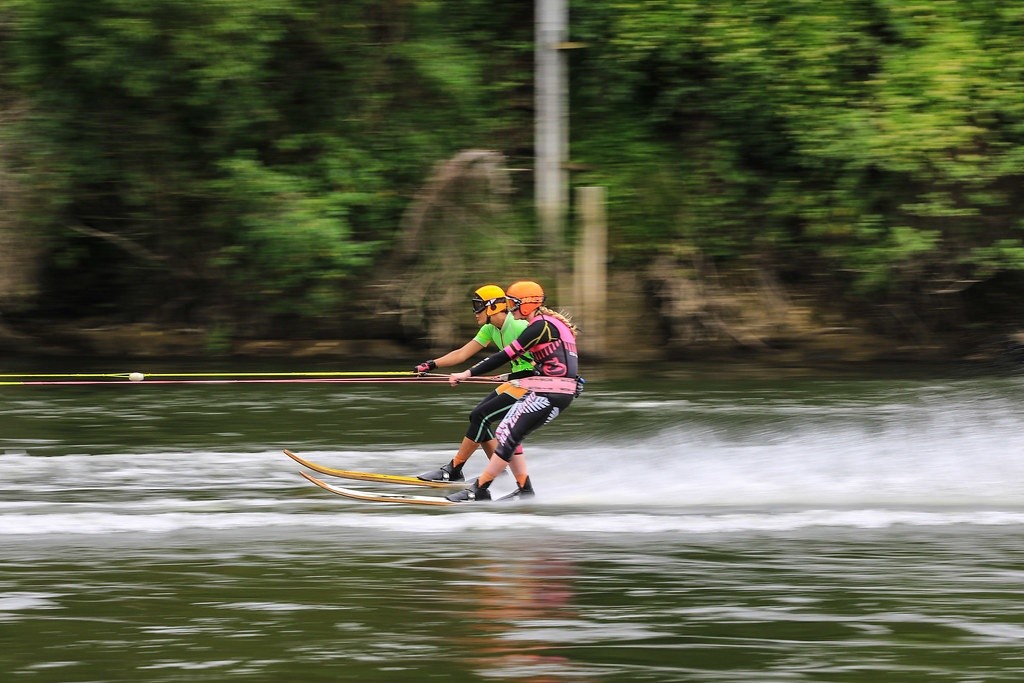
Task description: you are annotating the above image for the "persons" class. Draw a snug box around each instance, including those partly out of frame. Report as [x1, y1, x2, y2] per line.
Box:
[445, 281, 579, 503]
[413, 285, 535, 483]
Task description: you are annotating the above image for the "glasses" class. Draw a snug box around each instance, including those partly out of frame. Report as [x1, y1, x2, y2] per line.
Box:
[472, 296, 485, 314]
[504, 295, 521, 312]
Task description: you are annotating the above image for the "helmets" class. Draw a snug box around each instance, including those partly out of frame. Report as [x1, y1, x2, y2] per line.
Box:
[474, 285, 506, 316]
[505, 281, 544, 316]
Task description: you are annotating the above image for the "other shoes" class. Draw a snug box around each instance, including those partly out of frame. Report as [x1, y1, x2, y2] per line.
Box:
[445, 479, 493, 502]
[495, 475, 535, 502]
[417, 459, 466, 484]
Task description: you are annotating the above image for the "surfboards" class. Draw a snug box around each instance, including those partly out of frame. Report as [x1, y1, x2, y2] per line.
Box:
[282, 448, 475, 506]
